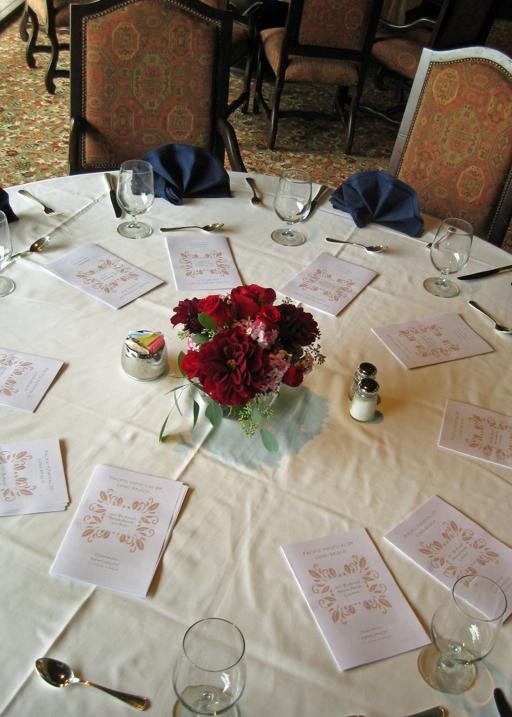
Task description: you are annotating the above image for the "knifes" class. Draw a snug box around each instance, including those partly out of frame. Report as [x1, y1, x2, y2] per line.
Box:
[490, 685, 511, 715]
[455, 263, 511, 283]
[301, 182, 329, 220]
[102, 169, 122, 218]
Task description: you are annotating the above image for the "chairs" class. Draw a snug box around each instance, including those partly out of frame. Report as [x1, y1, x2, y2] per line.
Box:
[19, 1, 69, 95]
[340, 0, 504, 136]
[255, 2, 382, 156]
[367, 39, 512, 252]
[68, 1, 249, 174]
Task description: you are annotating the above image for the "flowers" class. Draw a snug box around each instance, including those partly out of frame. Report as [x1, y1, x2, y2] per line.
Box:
[163, 275, 322, 437]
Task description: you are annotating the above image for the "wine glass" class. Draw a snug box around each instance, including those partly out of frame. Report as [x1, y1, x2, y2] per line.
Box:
[271, 168, 309, 248]
[0, 210, 17, 298]
[169, 617, 245, 717]
[113, 159, 156, 240]
[421, 217, 474, 295]
[415, 574, 507, 695]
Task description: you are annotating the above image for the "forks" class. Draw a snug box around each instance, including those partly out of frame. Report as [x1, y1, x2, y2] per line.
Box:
[19, 186, 61, 218]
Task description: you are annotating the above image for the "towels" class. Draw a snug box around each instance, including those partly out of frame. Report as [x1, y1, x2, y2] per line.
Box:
[319, 160, 428, 240]
[128, 135, 235, 209]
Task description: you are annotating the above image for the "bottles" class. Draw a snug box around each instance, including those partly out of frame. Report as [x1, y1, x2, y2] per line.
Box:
[348, 379, 380, 421]
[349, 359, 376, 403]
[122, 328, 169, 380]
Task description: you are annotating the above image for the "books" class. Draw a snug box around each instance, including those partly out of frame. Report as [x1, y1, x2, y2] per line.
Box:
[281, 527, 432, 672]
[278, 250, 378, 317]
[371, 312, 494, 371]
[0, 438, 70, 516]
[49, 464, 189, 598]
[163, 235, 244, 293]
[437, 397, 511, 470]
[387, 495, 512, 626]
[43, 243, 164, 309]
[0, 347, 64, 414]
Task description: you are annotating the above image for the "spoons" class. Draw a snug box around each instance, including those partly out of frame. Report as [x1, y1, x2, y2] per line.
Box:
[323, 235, 389, 255]
[467, 298, 509, 336]
[244, 174, 263, 207]
[158, 220, 226, 232]
[10, 234, 53, 263]
[30, 655, 153, 714]
